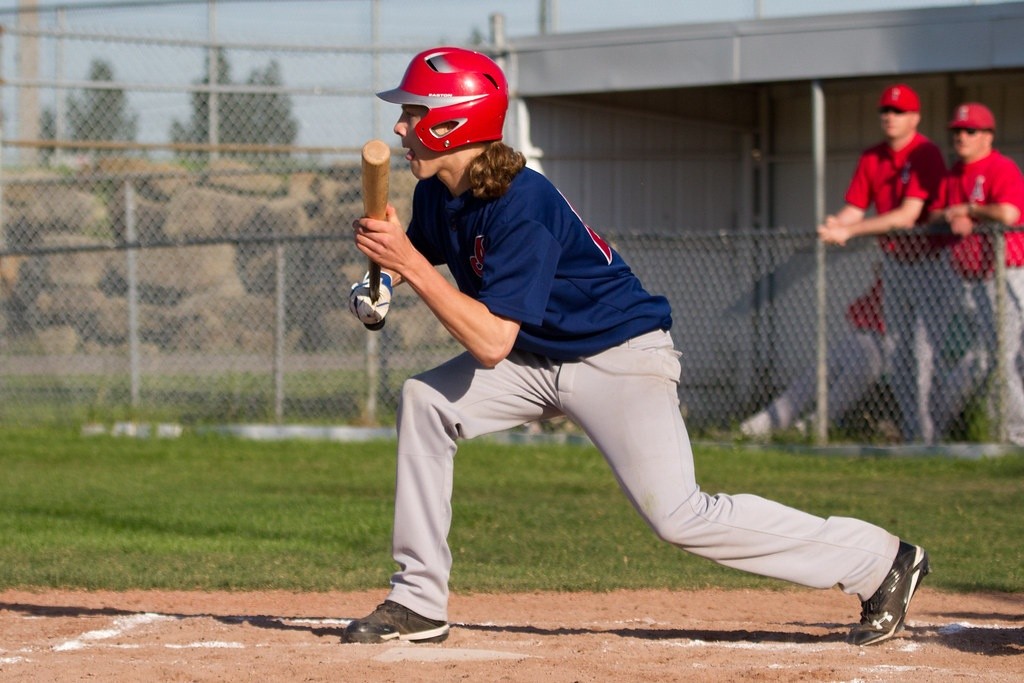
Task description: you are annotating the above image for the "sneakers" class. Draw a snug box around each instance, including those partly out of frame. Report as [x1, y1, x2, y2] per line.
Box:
[342, 599, 450, 644]
[847, 539, 929, 647]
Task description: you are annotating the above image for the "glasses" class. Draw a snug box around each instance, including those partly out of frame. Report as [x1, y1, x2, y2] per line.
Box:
[877, 107, 904, 115]
[953, 127, 992, 135]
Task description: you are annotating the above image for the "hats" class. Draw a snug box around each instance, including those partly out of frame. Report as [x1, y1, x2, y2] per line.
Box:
[878, 84, 921, 113]
[948, 102, 995, 130]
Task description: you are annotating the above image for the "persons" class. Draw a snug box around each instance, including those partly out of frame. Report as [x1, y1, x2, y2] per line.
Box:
[739, 244, 886, 440]
[815, 84, 968, 445]
[927, 103, 1023, 444]
[340, 47, 928, 646]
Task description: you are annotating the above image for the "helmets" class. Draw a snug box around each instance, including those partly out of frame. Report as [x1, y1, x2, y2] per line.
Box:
[375, 46, 508, 153]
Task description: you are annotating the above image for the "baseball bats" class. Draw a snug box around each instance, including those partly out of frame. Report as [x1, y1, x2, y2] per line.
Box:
[361, 139, 392, 331]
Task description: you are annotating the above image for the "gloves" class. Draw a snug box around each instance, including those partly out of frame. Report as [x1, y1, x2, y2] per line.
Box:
[349, 268, 392, 324]
